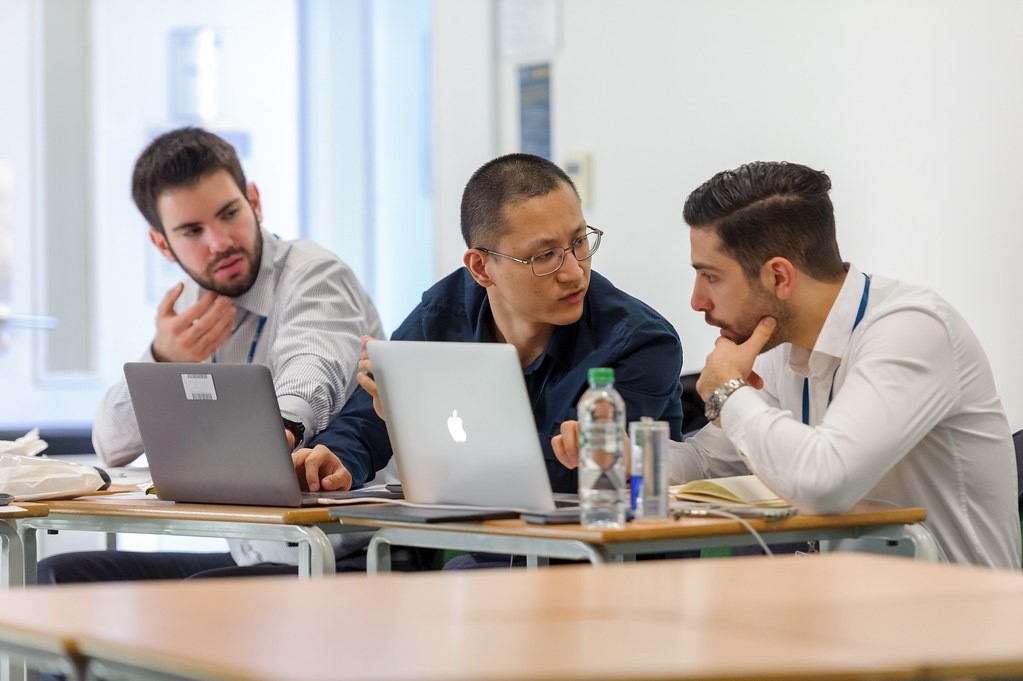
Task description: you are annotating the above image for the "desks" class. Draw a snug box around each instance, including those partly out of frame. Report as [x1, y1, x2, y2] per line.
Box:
[0, 470, 1023, 681]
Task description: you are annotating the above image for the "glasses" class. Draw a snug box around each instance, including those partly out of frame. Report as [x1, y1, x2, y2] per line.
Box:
[474, 225, 604, 277]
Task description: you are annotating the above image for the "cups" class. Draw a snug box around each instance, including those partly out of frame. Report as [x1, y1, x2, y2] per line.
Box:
[628, 418, 672, 526]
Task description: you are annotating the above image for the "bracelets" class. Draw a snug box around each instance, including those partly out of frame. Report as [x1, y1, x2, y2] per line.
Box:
[274, 412, 307, 449]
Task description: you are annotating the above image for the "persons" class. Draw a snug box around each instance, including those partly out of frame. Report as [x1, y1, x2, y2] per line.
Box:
[34, 125, 406, 589]
[551, 158, 1022, 583]
[282, 152, 685, 588]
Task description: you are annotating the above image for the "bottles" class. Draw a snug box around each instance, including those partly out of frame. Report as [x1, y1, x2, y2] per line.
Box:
[575, 366, 627, 531]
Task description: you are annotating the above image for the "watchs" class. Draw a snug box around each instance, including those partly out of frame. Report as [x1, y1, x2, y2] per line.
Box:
[704, 377, 748, 428]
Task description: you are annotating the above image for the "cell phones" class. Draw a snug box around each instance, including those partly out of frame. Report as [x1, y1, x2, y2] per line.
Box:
[671, 503, 797, 521]
[521, 511, 636, 525]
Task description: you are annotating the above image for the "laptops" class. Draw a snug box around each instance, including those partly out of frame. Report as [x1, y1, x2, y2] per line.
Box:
[367, 339, 585, 517]
[122, 363, 404, 508]
